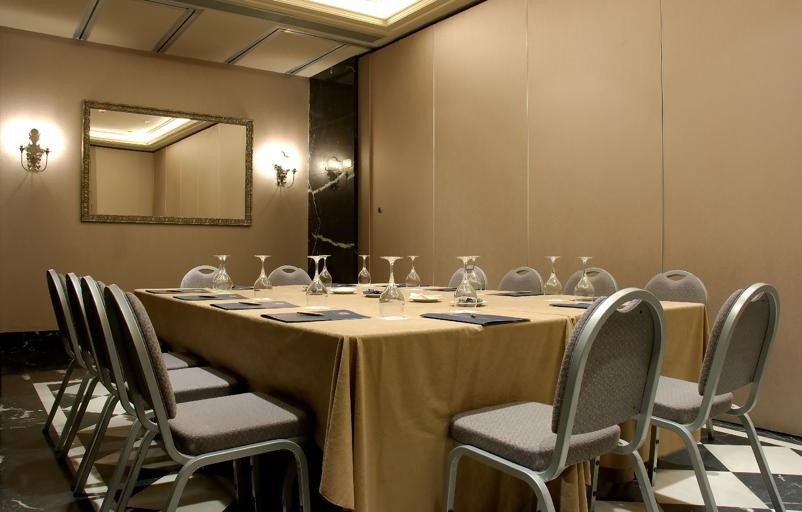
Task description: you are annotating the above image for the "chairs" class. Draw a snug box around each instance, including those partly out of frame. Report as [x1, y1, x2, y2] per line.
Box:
[73, 275, 242, 512]
[59, 272, 241, 490]
[180, 265, 232, 289]
[585, 283, 786, 512]
[42, 268, 202, 452]
[443, 287, 668, 512]
[448, 265, 488, 290]
[498, 267, 543, 292]
[564, 267, 618, 297]
[268, 265, 312, 285]
[644, 270, 708, 305]
[104, 283, 310, 512]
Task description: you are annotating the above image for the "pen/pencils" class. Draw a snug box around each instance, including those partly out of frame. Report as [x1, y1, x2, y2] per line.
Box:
[199, 296, 217, 298]
[297, 312, 325, 316]
[166, 290, 182, 292]
[470, 313, 476, 318]
[239, 302, 261, 305]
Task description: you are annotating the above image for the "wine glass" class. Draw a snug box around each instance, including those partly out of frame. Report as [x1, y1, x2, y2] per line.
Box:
[210, 253, 233, 296]
[542, 253, 563, 302]
[573, 252, 597, 303]
[357, 254, 372, 287]
[377, 255, 406, 316]
[404, 255, 422, 288]
[305, 255, 329, 313]
[319, 254, 333, 288]
[453, 256, 482, 307]
[252, 255, 276, 303]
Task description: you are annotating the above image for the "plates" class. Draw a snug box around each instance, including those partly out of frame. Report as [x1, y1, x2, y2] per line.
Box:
[329, 285, 356, 297]
[331, 287, 488, 306]
[409, 293, 440, 303]
[362, 288, 382, 298]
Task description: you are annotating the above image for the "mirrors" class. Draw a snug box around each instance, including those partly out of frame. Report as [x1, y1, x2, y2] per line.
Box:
[80, 99, 254, 226]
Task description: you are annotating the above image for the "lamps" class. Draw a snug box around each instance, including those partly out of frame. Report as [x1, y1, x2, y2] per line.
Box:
[7, 126, 64, 174]
[264, 144, 302, 188]
